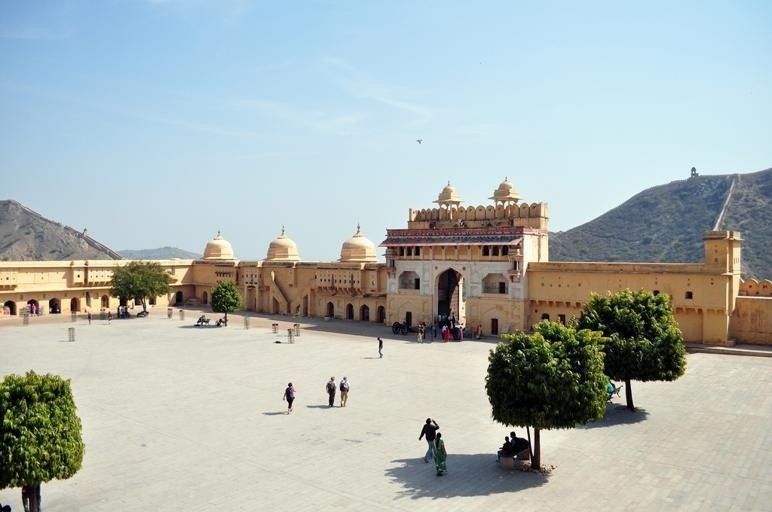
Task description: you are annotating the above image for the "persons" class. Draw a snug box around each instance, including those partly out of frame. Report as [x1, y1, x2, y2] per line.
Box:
[418, 417, 439, 463]
[430, 432, 448, 477]
[324, 375, 336, 408]
[416, 311, 483, 344]
[496, 434, 513, 461]
[337, 376, 350, 408]
[375, 336, 384, 358]
[282, 382, 296, 414]
[509, 430, 522, 455]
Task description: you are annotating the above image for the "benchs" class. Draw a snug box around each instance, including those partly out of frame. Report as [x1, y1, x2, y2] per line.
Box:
[607, 385, 623, 404]
[502, 448, 529, 469]
